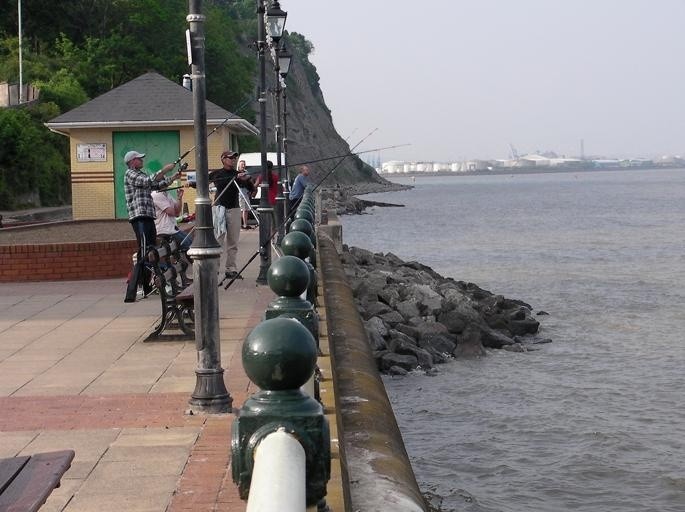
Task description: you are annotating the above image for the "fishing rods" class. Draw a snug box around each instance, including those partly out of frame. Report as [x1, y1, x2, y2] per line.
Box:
[169, 95, 259, 173]
[218, 126, 380, 291]
[155, 144, 412, 194]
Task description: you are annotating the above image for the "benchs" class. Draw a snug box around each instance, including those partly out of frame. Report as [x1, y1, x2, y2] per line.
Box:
[0, 450, 75, 512]
[139, 241, 195, 344]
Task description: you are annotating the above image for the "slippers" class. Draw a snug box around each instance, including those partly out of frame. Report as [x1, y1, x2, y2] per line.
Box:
[242, 225, 252, 229]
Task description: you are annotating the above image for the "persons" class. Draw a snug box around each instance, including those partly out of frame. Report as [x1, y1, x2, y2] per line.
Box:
[237, 159, 258, 230]
[150, 173, 194, 286]
[252, 160, 280, 233]
[285, 163, 310, 236]
[122, 150, 183, 304]
[185, 151, 257, 281]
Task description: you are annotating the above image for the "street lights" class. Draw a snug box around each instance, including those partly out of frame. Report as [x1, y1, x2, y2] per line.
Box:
[264, 1, 292, 243]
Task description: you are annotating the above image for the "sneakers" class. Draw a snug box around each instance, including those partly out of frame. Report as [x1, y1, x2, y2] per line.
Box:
[226, 271, 241, 278]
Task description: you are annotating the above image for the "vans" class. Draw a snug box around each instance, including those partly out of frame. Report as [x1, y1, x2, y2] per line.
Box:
[236, 152, 285, 226]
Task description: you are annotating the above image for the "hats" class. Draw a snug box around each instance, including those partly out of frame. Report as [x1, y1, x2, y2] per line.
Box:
[124, 151, 145, 163]
[221, 151, 238, 160]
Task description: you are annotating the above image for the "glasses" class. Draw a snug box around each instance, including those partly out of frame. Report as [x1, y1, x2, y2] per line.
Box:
[223, 157, 237, 159]
